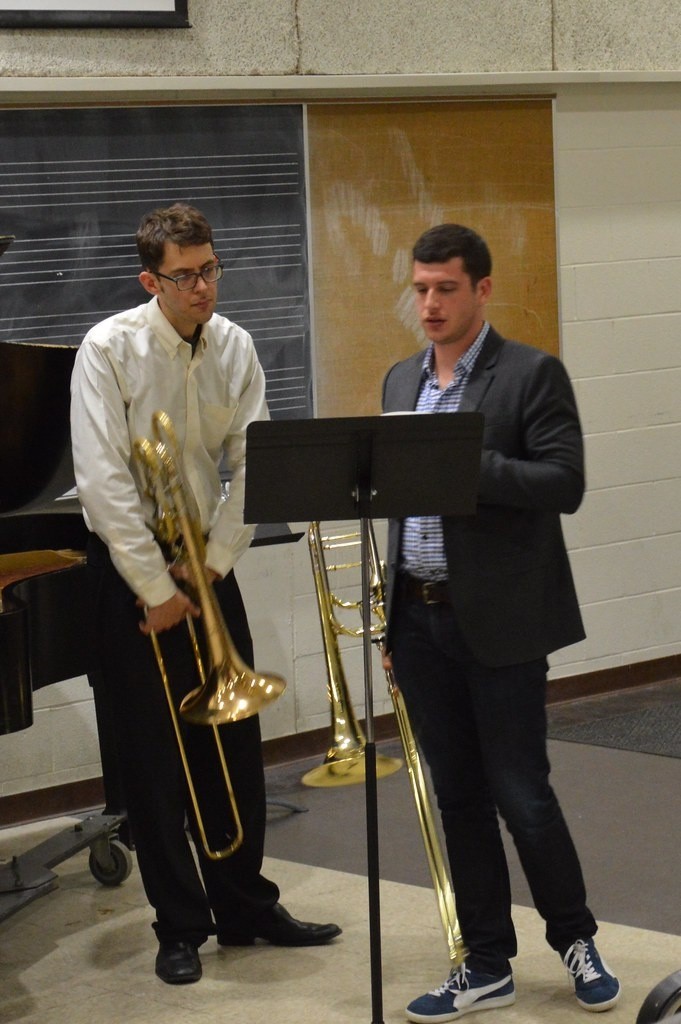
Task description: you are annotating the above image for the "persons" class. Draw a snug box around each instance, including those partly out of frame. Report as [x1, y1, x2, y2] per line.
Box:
[70, 205, 343, 984]
[381, 223, 621, 1024]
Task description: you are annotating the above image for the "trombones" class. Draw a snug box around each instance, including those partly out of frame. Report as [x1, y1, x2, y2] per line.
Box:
[300, 518, 469, 967]
[130, 409, 292, 863]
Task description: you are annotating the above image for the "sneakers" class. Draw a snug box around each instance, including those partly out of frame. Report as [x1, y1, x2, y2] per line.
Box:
[559, 934, 622, 1012]
[406, 961, 518, 1023]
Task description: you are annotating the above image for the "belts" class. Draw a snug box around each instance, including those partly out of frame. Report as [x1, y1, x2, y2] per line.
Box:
[396, 573, 453, 606]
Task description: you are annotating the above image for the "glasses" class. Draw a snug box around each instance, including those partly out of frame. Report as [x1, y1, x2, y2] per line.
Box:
[151, 251, 225, 293]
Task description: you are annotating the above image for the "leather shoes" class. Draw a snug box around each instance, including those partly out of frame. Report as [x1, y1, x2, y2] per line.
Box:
[218, 899, 345, 948]
[153, 927, 204, 984]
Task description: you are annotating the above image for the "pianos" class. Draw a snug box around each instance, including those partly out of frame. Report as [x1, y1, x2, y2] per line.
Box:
[0, 338, 138, 926]
